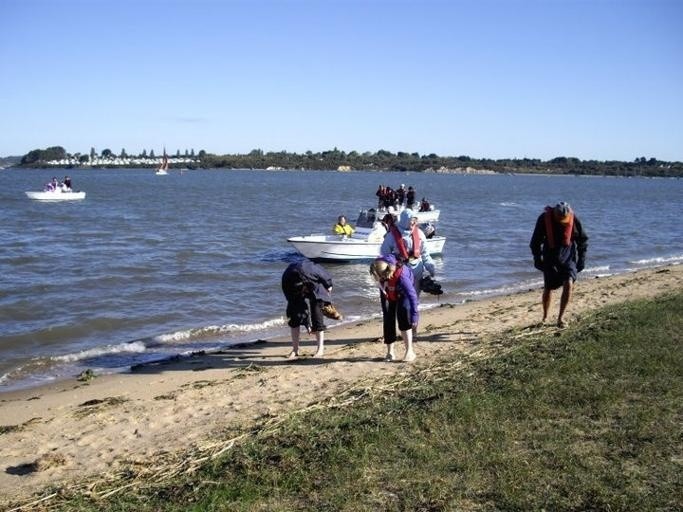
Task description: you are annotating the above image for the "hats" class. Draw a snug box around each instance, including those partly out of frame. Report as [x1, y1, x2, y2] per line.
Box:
[555, 201, 571, 218]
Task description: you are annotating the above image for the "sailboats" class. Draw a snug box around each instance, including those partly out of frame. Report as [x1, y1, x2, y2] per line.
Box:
[154, 156, 169, 175]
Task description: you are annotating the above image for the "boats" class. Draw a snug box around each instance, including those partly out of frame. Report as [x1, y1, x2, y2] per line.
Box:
[24, 183, 86, 202]
[287, 204, 446, 263]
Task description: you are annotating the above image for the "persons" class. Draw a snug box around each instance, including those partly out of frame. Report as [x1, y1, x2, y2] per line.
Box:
[419, 198, 432, 212]
[370, 255, 417, 364]
[355, 208, 376, 229]
[375, 185, 388, 211]
[380, 213, 398, 238]
[380, 208, 437, 299]
[333, 215, 356, 237]
[280, 259, 333, 358]
[45, 177, 57, 192]
[382, 187, 398, 212]
[529, 201, 589, 329]
[396, 184, 408, 209]
[63, 176, 72, 192]
[407, 186, 416, 210]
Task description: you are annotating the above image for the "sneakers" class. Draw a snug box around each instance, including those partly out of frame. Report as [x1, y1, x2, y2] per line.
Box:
[321, 302, 343, 321]
[419, 273, 444, 295]
